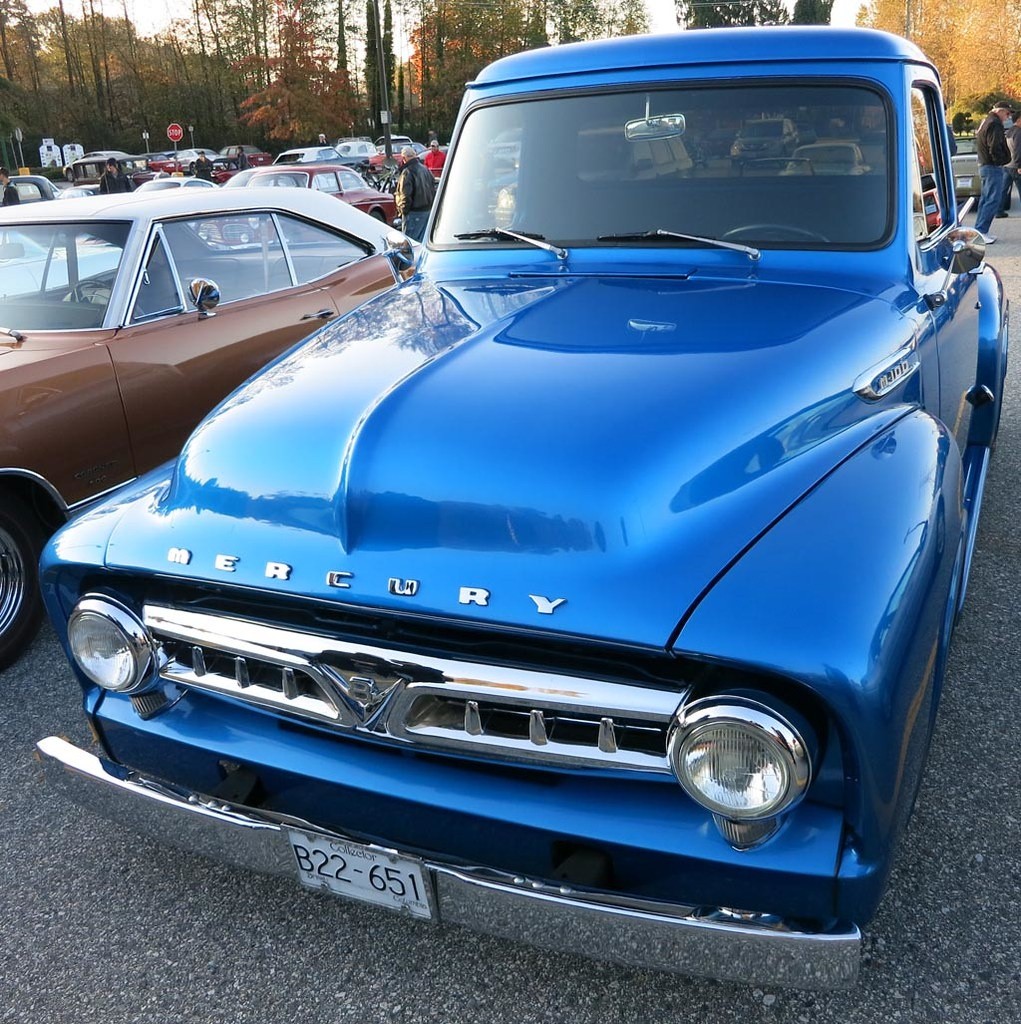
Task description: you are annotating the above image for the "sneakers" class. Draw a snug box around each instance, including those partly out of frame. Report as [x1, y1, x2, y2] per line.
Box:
[981, 234, 998, 244]
[995, 211, 1009, 218]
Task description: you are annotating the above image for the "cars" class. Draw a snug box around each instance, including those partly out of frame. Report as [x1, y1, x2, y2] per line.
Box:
[0, 133, 522, 244]
[0, 186, 425, 673]
[491, 122, 695, 242]
[949, 135, 982, 201]
[728, 117, 800, 172]
[24, 19, 1014, 996]
[776, 141, 872, 182]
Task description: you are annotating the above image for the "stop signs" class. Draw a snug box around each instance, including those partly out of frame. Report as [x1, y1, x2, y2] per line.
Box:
[167, 123, 184, 142]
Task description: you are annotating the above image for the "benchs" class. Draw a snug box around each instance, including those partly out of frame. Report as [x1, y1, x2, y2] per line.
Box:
[527, 173, 888, 246]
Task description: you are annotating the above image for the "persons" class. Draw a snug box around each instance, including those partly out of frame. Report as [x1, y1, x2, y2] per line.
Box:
[997, 110, 1021, 219]
[396, 145, 436, 243]
[194, 151, 213, 181]
[237, 146, 247, 171]
[974, 102, 1015, 245]
[99, 158, 132, 194]
[0, 167, 20, 206]
[319, 134, 330, 146]
[424, 141, 446, 178]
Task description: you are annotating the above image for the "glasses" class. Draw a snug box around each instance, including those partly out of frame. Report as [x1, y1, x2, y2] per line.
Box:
[1003, 109, 1010, 115]
[432, 145, 438, 147]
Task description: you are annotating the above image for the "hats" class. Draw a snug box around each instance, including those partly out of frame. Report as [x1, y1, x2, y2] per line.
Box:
[105, 157, 117, 167]
[198, 151, 205, 154]
[430, 139, 439, 146]
[994, 101, 1011, 109]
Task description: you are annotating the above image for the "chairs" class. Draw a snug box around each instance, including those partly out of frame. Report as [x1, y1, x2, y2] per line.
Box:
[271, 256, 363, 290]
[159, 256, 245, 311]
